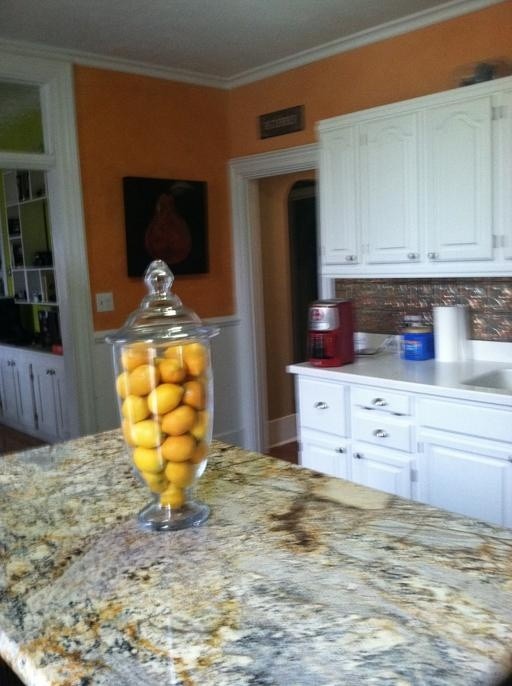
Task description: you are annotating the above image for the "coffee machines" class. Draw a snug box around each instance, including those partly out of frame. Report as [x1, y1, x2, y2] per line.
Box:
[308, 298, 354, 367]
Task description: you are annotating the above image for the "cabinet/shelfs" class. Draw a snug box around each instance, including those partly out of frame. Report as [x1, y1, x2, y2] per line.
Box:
[0, 162, 59, 308]
[33, 347, 66, 445]
[286, 355, 512, 527]
[0, 344, 36, 438]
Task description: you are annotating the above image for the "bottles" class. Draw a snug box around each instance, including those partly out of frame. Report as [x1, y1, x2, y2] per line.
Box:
[399, 313, 434, 361]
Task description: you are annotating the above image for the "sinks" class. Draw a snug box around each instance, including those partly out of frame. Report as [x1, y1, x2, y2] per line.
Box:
[462, 369, 512, 389]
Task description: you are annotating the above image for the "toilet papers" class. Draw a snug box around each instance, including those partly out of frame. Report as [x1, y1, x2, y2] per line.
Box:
[432, 304, 458, 363]
[458, 307, 468, 363]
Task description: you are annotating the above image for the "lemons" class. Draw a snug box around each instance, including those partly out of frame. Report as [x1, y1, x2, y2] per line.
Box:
[116, 337, 211, 511]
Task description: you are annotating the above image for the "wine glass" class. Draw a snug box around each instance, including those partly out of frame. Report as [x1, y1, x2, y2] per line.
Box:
[103, 323, 223, 529]
